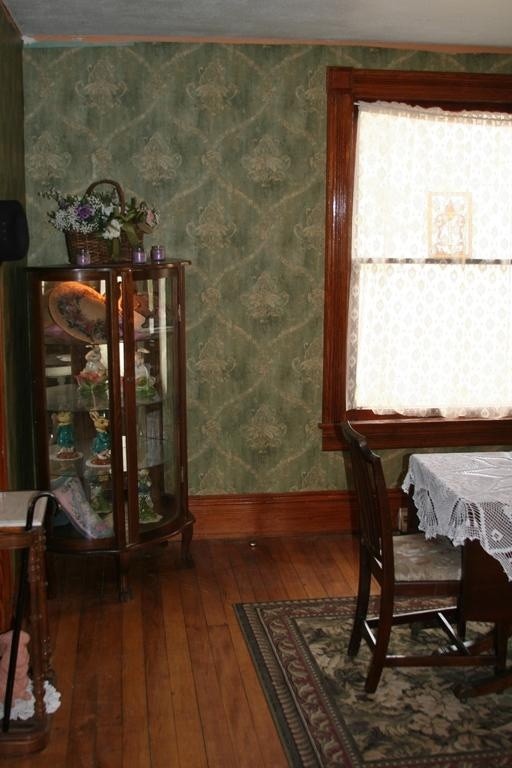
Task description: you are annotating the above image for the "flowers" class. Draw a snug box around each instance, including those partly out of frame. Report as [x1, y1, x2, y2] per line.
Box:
[36, 184, 160, 264]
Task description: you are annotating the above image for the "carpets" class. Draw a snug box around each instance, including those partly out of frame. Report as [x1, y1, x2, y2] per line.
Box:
[230, 592, 512, 768]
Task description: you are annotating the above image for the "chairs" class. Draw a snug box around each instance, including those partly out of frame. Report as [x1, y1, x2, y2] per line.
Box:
[338, 419, 508, 701]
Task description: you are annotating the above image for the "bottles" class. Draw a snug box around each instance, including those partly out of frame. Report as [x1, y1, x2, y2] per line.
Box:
[132, 246, 145, 264]
[151, 245, 165, 261]
[76, 248, 91, 265]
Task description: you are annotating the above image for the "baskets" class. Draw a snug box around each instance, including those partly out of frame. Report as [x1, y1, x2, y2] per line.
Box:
[62, 179, 146, 266]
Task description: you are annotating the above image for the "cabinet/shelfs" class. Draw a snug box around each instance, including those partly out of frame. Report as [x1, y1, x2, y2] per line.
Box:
[14, 257, 196, 602]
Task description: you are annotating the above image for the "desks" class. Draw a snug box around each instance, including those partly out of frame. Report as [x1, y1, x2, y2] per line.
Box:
[402, 449, 512, 695]
[1, 490, 55, 754]
